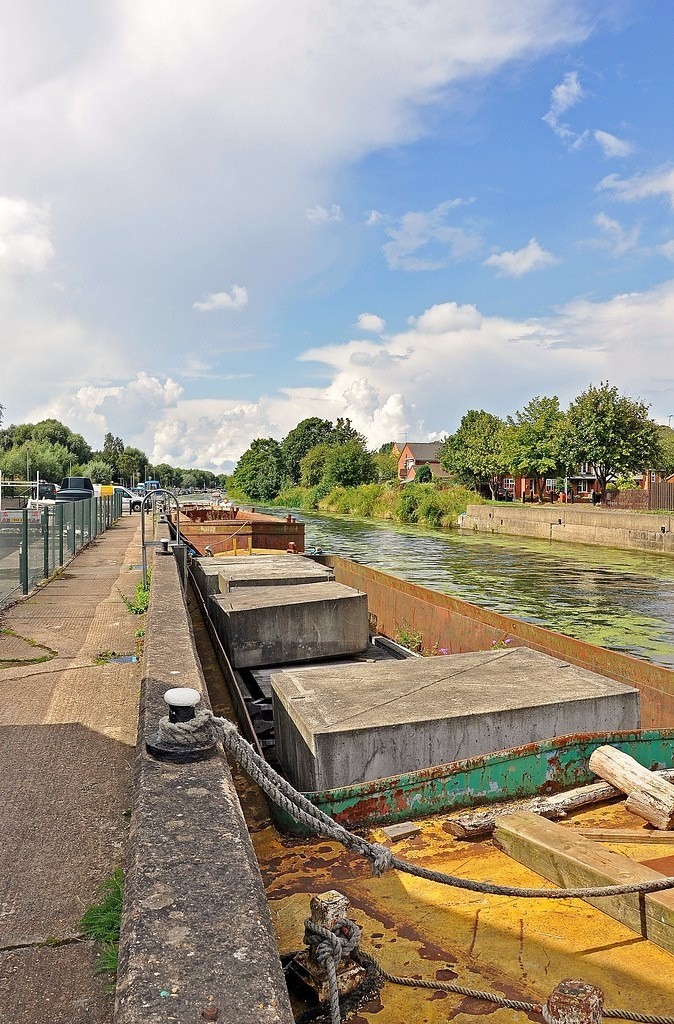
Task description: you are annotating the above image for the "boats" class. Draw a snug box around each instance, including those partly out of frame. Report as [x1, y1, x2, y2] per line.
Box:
[210, 489, 221, 497]
[216, 498, 232, 508]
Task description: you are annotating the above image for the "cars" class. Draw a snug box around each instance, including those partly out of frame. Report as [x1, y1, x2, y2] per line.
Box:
[25, 476, 212, 512]
[494, 488, 513, 502]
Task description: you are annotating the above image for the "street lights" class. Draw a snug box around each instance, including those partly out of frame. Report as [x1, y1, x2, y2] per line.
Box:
[133, 473, 136, 488]
[69, 455, 80, 476]
[143, 465, 147, 482]
